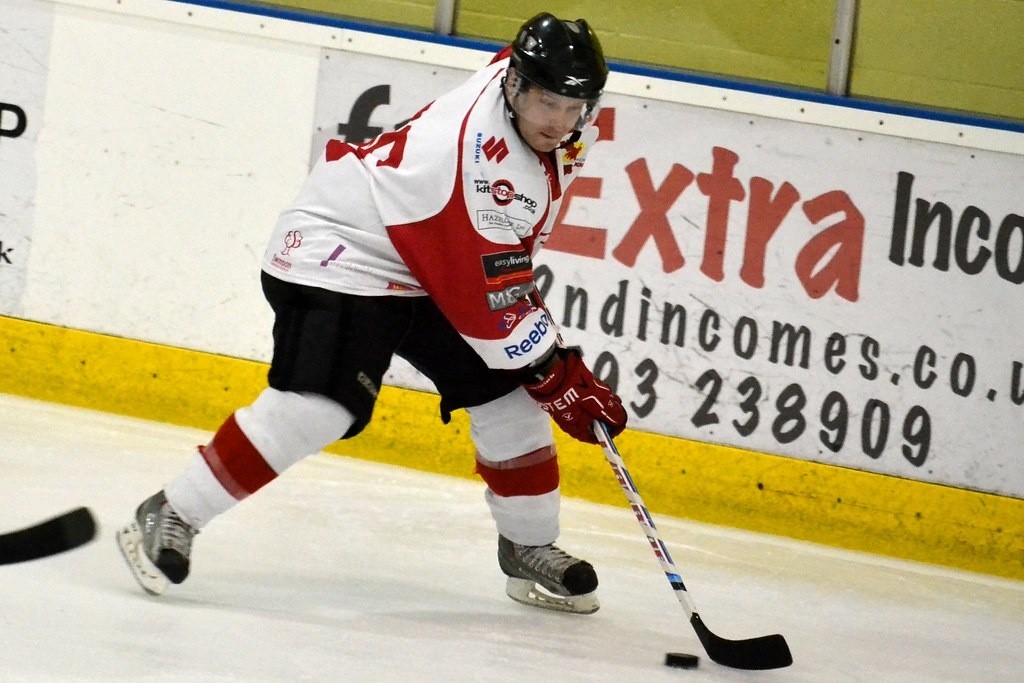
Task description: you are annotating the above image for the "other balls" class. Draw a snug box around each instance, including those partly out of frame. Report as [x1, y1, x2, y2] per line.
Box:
[664, 650, 698, 670]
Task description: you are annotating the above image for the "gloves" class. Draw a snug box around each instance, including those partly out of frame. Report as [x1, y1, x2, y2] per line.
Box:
[521, 343, 628, 445]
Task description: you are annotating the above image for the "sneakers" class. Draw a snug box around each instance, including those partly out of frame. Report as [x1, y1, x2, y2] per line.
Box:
[498, 533, 601, 615]
[115, 489, 201, 599]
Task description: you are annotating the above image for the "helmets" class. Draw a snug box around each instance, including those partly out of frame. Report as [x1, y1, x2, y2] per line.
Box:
[509, 11, 609, 100]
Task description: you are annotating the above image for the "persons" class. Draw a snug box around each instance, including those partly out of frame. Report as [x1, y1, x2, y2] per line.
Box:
[116, 11, 628, 615]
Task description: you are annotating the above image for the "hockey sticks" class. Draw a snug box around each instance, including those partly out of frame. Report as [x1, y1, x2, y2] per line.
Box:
[0, 500, 97, 571]
[526, 280, 796, 673]
